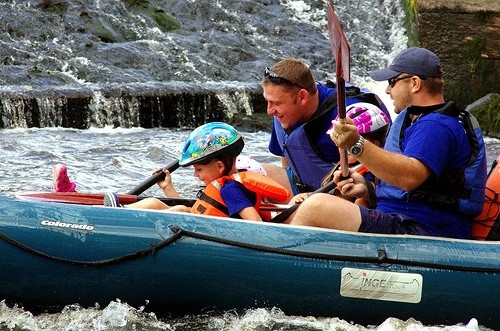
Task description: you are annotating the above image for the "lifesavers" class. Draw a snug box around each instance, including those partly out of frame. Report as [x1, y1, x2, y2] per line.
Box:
[471, 154, 500, 242]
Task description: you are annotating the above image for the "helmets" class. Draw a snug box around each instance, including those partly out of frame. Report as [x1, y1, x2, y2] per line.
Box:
[178, 121, 245, 167]
[325, 102, 390, 140]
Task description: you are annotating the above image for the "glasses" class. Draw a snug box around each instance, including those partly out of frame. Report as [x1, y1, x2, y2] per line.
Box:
[263, 67, 308, 92]
[388, 76, 427, 87]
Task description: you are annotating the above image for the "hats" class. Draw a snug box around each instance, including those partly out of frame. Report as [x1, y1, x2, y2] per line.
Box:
[371, 47, 442, 81]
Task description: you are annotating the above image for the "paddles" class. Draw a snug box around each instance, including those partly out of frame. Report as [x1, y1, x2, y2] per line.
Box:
[326, 3, 351, 180]
[266, 182, 337, 223]
[126, 159, 180, 196]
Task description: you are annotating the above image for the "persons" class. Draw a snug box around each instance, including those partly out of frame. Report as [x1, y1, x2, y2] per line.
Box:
[250, 59, 392, 203]
[103, 122, 289, 223]
[54, 103, 390, 225]
[284, 47, 486, 239]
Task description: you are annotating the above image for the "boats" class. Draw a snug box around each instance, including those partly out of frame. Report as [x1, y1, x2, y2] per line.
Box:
[0, 193, 500, 330]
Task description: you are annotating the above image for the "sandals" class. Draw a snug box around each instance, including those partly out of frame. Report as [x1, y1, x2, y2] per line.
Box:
[54, 163, 76, 192]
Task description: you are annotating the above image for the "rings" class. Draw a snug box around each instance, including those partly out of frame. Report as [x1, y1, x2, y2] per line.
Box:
[335, 133, 340, 139]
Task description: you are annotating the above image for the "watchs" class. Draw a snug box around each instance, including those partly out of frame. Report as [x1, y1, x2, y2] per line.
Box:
[350, 135, 364, 156]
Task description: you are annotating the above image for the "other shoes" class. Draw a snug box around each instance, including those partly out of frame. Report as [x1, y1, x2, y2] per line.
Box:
[104, 193, 124, 208]
